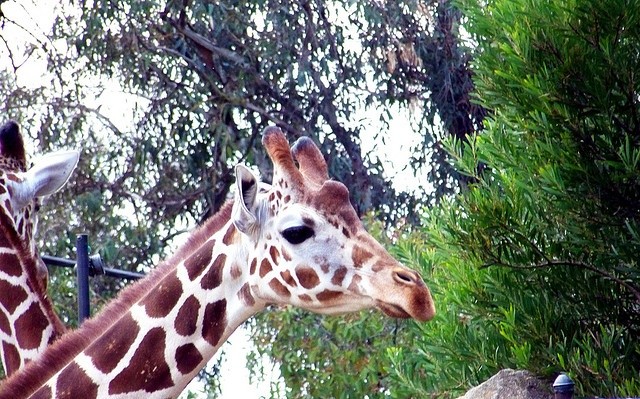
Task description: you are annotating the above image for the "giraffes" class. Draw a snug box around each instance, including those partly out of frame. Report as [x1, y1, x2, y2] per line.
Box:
[0, 120, 81, 381]
[0, 126, 435, 399]
